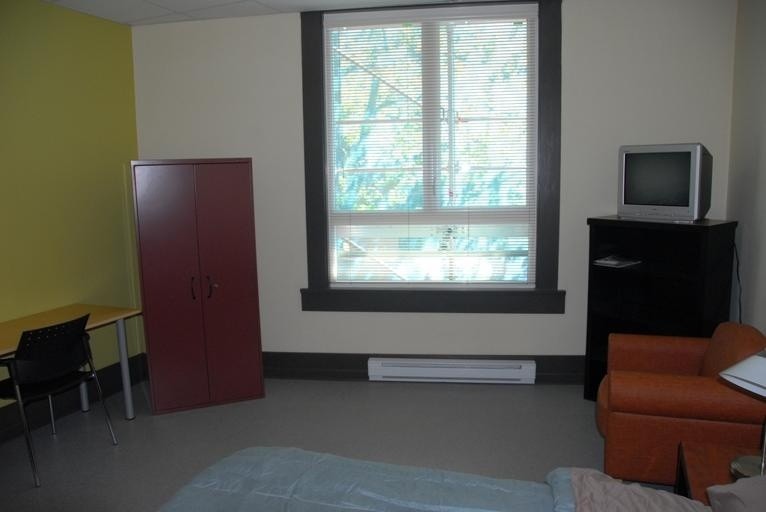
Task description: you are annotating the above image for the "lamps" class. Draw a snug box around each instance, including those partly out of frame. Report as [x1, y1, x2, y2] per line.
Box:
[730, 423, 766, 478]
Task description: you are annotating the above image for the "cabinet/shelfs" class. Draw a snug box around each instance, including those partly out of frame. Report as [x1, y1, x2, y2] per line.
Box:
[583, 215, 739, 402]
[130, 158, 267, 415]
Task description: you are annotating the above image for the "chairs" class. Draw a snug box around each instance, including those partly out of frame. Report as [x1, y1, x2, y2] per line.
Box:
[0, 312, 118, 488]
[595, 321, 766, 487]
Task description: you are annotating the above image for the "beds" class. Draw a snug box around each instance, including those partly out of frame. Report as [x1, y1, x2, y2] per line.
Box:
[161, 445, 766, 512]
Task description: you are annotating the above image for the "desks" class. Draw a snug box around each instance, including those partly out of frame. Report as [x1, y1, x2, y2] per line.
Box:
[0, 301, 141, 420]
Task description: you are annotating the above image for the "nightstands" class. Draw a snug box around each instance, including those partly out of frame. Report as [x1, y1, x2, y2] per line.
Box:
[674, 441, 766, 503]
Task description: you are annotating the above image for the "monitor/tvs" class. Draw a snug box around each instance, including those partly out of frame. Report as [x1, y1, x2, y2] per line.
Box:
[617, 143, 713, 224]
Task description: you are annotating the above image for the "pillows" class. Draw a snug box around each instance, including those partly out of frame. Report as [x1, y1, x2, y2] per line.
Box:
[706, 475, 766, 512]
